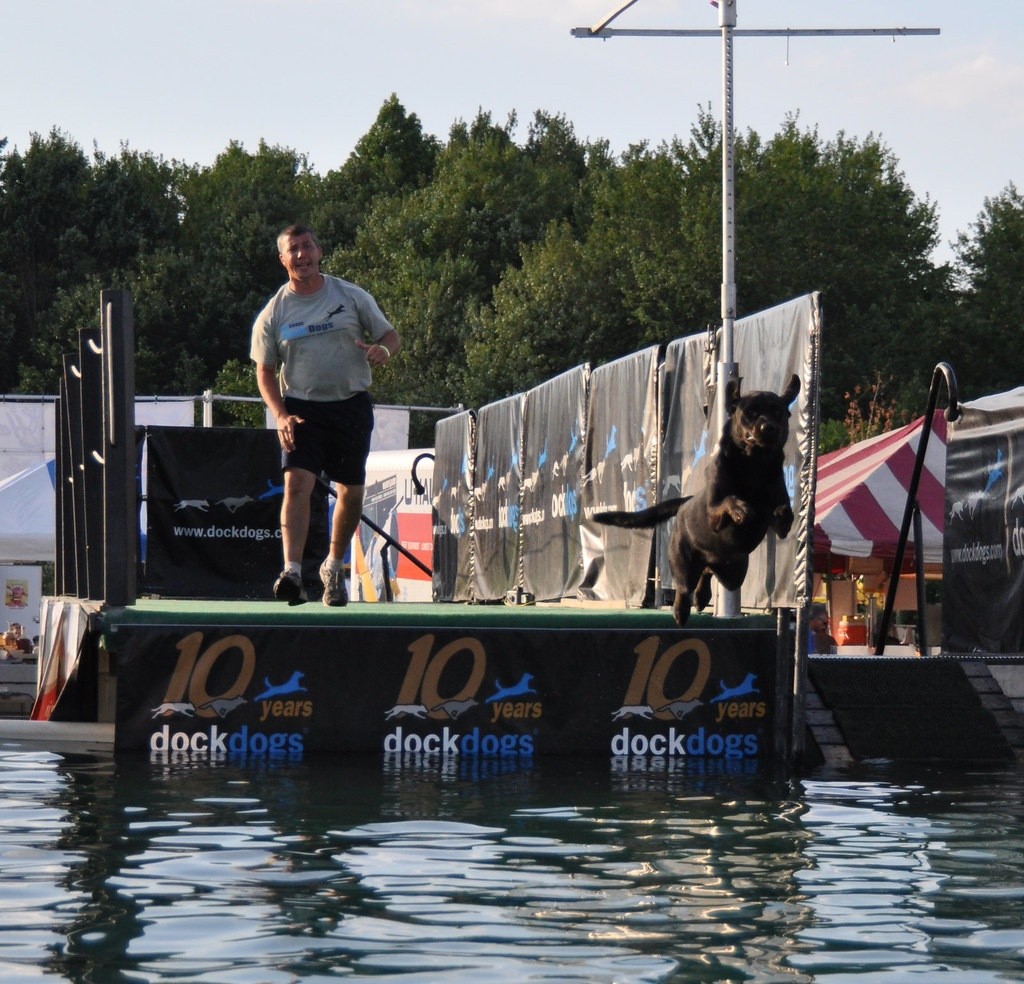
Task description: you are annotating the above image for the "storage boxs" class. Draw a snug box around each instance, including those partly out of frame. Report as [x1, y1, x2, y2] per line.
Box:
[840, 621, 866, 645]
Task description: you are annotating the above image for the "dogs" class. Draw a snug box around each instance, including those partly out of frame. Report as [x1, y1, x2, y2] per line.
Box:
[592, 372, 803, 627]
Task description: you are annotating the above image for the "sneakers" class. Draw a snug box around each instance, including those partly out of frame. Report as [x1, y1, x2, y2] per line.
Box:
[273, 569, 308, 606]
[320, 559, 348, 606]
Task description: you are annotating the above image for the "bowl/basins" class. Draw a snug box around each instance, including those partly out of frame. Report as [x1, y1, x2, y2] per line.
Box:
[9, 650, 24, 658]
[0, 650, 8, 660]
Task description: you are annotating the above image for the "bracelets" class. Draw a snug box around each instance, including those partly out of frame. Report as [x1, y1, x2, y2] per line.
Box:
[379, 345, 390, 368]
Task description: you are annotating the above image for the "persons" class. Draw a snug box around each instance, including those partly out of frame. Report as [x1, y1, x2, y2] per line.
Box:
[806, 602, 826, 653]
[812, 613, 838, 654]
[0, 623, 40, 661]
[250, 227, 401, 607]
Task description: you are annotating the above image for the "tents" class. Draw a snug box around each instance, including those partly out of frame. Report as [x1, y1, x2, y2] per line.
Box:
[0, 457, 56, 563]
[814, 409, 946, 646]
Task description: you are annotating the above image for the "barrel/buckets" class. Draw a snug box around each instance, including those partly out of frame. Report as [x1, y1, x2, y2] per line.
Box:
[836, 621, 866, 646]
